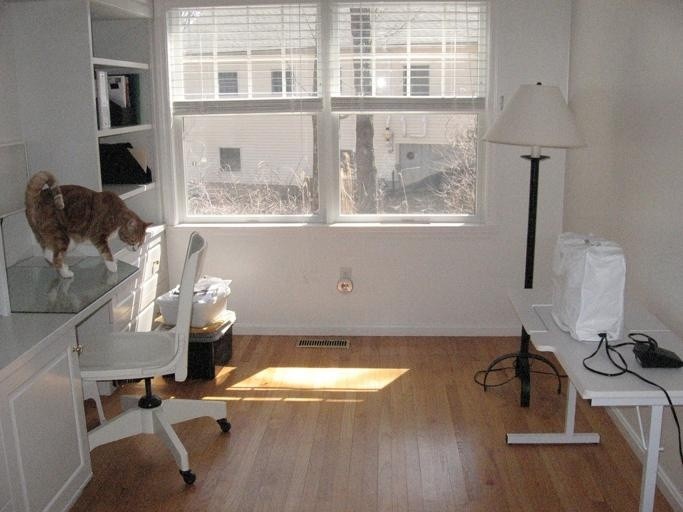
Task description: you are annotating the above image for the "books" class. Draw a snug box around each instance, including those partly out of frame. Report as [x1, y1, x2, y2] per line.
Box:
[94, 67, 142, 132]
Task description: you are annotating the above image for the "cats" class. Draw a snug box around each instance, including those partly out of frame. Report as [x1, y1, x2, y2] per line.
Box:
[23, 170, 155, 279]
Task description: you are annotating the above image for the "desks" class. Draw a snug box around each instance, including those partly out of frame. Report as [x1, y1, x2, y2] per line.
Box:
[504, 282, 683, 512]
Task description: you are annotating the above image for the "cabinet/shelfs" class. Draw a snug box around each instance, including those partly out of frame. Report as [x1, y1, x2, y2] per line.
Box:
[1, 0, 169, 512]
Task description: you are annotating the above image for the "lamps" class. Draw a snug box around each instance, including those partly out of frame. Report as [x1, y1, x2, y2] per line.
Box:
[480, 79, 588, 409]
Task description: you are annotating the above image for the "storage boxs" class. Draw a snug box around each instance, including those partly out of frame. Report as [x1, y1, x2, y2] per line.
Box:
[155, 282, 230, 329]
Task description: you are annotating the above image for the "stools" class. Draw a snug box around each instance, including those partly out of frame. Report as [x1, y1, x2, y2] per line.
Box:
[150, 309, 238, 381]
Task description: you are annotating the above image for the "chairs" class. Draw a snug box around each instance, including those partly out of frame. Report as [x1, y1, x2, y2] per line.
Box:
[75, 228, 231, 488]
